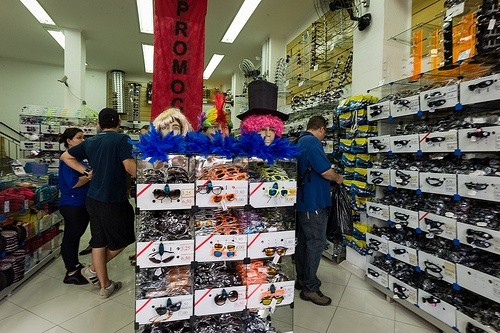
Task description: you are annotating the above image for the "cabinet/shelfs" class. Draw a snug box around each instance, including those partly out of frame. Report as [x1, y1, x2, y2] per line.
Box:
[0, 47, 500, 333]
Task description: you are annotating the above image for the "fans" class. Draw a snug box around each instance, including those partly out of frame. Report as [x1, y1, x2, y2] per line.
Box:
[238, 59, 262, 81]
[312, 0, 373, 37]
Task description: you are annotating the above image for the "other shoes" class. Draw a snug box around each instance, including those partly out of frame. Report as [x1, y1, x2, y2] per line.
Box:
[79, 248, 92, 255]
[338, 101, 378, 255]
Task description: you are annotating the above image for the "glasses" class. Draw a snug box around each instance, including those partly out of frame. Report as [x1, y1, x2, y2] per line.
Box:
[137, 163, 295, 333]
[133, 92, 139, 116]
[367, 72, 500, 333]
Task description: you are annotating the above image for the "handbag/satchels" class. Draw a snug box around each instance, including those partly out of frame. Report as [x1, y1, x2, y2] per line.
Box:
[326, 182, 353, 245]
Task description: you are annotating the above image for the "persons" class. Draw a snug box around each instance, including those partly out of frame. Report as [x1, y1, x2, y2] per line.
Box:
[295, 115, 343, 306]
[200, 108, 233, 141]
[153, 107, 193, 138]
[236, 81, 290, 147]
[57, 108, 136, 299]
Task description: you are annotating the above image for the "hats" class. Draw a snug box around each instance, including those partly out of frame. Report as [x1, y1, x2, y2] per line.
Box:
[236, 79, 289, 121]
[98, 107, 119, 123]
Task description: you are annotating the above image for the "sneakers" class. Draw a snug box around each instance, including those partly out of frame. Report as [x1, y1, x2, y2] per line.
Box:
[63, 262, 89, 284]
[295, 281, 331, 306]
[99, 280, 122, 299]
[80, 264, 100, 287]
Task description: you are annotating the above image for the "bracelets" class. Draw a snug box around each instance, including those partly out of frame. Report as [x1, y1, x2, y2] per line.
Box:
[83, 168, 91, 177]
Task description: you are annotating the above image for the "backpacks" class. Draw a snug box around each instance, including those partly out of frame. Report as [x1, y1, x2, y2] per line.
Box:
[291, 134, 310, 202]
